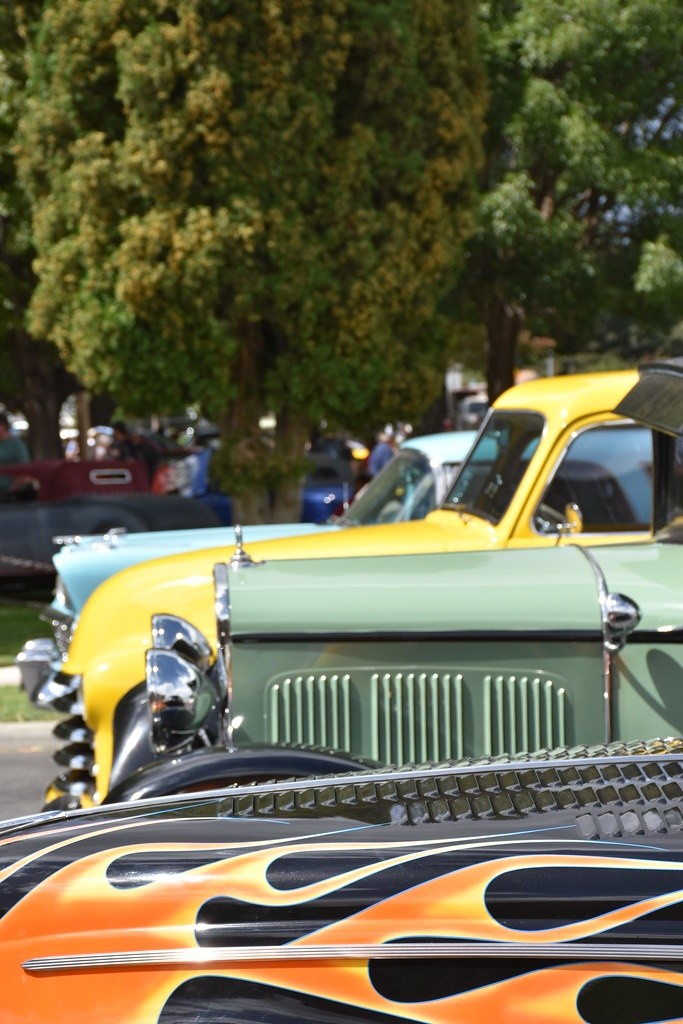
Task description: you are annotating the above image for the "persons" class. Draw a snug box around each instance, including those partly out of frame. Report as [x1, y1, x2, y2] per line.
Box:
[112, 422, 158, 486]
[322, 432, 344, 452]
[0, 414, 32, 491]
[369, 432, 394, 474]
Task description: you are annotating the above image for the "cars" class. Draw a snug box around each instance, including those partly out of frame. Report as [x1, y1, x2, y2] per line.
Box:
[108, 353, 682, 786]
[43, 367, 644, 802]
[13, 427, 557, 714]
[0, 734, 682, 1022]
